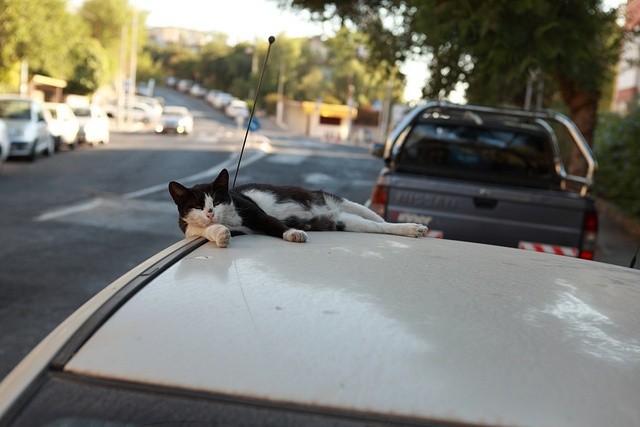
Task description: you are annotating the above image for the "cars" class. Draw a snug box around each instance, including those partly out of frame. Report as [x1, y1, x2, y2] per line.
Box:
[44, 103, 79, 151]
[1, 94, 55, 163]
[158, 105, 193, 135]
[65, 101, 109, 145]
[103, 102, 158, 126]
[0, 229, 639, 427]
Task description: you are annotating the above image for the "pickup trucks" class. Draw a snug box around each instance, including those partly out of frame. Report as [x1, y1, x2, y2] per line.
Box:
[366, 98, 601, 260]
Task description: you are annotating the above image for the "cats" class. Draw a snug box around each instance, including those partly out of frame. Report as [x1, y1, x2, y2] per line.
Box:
[168, 168, 429, 248]
